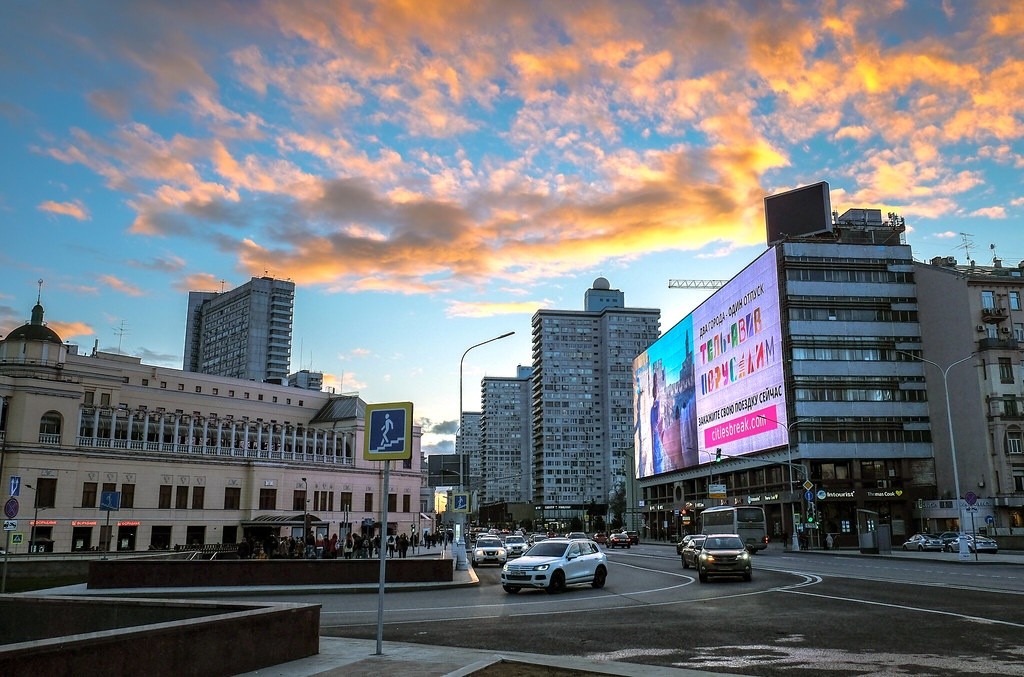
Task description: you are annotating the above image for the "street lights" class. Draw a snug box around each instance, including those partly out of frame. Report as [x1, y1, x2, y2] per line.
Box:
[895, 349, 984, 558]
[454, 331, 517, 570]
[301, 477, 308, 549]
[24, 483, 51, 553]
[685, 446, 715, 507]
[756, 414, 814, 552]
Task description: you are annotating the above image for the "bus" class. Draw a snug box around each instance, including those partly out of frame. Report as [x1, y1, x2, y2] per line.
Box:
[699, 505, 769, 555]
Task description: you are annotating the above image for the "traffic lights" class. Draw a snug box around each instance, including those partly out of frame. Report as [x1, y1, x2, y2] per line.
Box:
[807, 508, 813, 523]
[817, 510, 822, 523]
[716, 447, 722, 463]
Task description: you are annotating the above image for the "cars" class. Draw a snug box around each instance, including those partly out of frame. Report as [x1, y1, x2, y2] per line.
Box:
[680, 537, 706, 570]
[472, 526, 589, 559]
[939, 531, 962, 549]
[593, 533, 608, 544]
[621, 530, 639, 545]
[946, 533, 998, 554]
[901, 533, 943, 551]
[605, 533, 632, 549]
[676, 534, 705, 555]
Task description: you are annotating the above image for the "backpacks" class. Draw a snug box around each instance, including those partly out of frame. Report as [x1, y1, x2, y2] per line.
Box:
[347, 540, 352, 548]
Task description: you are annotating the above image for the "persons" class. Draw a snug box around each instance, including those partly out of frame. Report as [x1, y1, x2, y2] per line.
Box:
[237, 526, 453, 559]
[782, 531, 788, 548]
[825, 534, 840, 551]
[799, 533, 806, 551]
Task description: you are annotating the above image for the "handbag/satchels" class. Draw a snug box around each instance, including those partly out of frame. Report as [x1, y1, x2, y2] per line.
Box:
[335, 542, 339, 550]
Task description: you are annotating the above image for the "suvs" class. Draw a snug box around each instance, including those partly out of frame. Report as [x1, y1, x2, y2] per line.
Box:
[693, 533, 754, 583]
[471, 537, 508, 567]
[500, 538, 609, 596]
